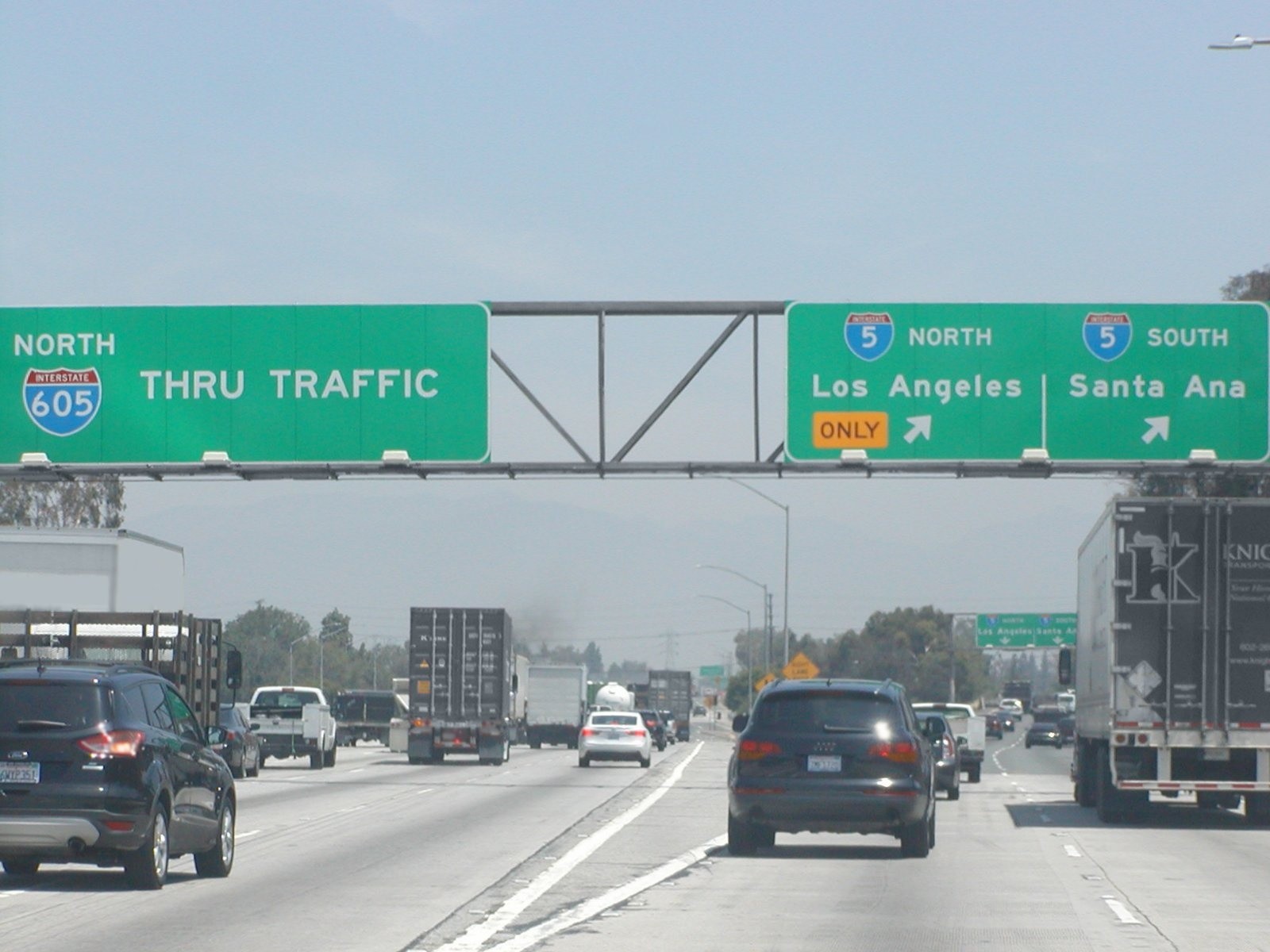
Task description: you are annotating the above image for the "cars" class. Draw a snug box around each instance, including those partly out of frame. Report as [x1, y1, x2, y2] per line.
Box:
[998, 712, 1016, 731]
[999, 698, 1023, 719]
[980, 713, 1003, 740]
[1025, 721, 1063, 749]
[575, 704, 651, 770]
[911, 710, 969, 801]
[691, 706, 707, 716]
[217, 702, 264, 779]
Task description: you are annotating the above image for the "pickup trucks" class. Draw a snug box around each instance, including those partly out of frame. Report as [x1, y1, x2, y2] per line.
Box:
[243, 686, 339, 769]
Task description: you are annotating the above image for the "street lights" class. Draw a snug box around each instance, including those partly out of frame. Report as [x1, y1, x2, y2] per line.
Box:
[696, 563, 769, 676]
[289, 621, 342, 687]
[319, 626, 350, 691]
[697, 594, 754, 716]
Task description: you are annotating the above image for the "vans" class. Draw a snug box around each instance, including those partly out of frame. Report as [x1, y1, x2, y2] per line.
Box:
[1034, 691, 1075, 736]
[586, 705, 614, 724]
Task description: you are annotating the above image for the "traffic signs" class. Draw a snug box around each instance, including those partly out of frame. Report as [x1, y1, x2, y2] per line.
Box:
[784, 299, 1270, 480]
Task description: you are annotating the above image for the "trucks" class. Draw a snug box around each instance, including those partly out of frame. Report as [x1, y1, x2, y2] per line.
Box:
[1058, 493, 1270, 823]
[647, 669, 694, 742]
[1, 603, 246, 749]
[405, 605, 517, 766]
[1000, 679, 1036, 714]
[0, 523, 200, 659]
[909, 702, 987, 784]
[521, 662, 589, 750]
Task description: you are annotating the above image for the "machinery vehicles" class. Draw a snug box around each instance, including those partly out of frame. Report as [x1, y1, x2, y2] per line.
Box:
[593, 681, 636, 714]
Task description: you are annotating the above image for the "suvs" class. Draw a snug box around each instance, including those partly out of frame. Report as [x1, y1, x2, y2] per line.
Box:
[726, 677, 945, 860]
[656, 709, 679, 745]
[630, 709, 668, 752]
[0, 658, 237, 891]
[335, 690, 410, 748]
